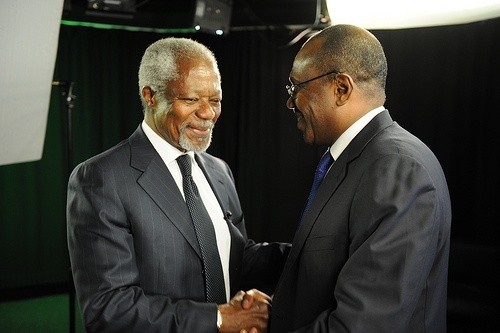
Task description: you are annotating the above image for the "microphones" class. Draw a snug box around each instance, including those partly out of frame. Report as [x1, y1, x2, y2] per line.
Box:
[226, 210, 232, 218]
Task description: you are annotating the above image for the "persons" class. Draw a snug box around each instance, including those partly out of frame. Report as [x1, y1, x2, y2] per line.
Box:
[67, 36, 293, 332]
[239, 22, 452, 333]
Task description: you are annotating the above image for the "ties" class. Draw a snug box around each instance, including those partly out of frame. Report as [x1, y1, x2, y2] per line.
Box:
[297, 152, 335, 235]
[175, 153, 229, 304]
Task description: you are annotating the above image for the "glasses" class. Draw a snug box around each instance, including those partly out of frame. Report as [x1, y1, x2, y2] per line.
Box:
[285, 68, 339, 99]
